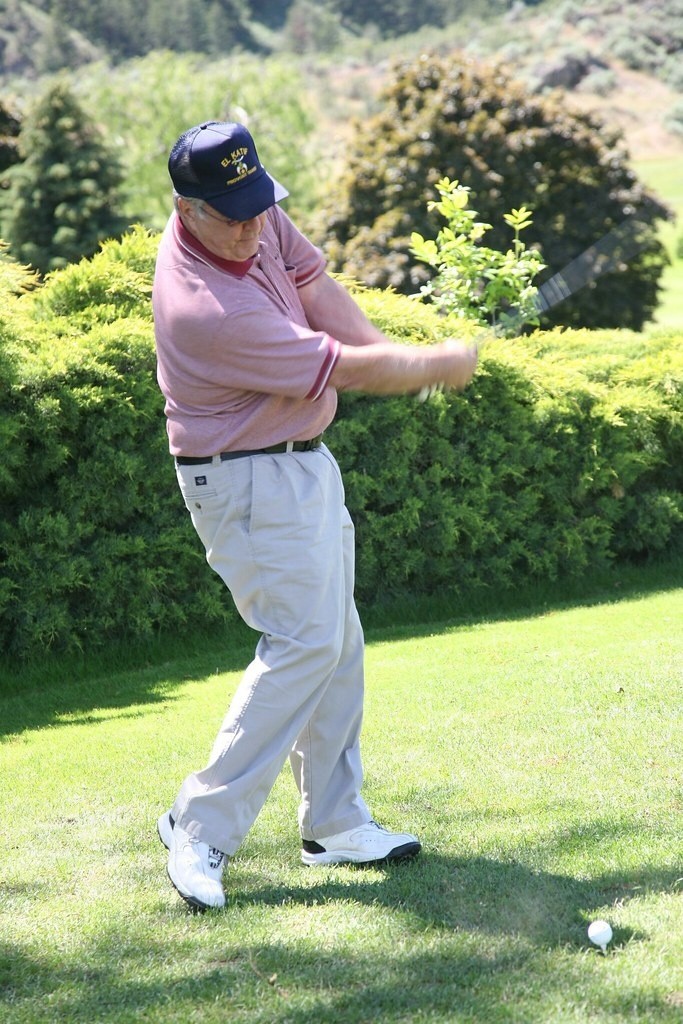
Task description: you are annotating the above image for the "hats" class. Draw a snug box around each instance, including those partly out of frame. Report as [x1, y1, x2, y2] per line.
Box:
[168, 121, 289, 222]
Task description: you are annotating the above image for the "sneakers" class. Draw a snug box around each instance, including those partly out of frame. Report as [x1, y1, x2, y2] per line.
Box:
[302, 820, 423, 866]
[156, 808, 225, 911]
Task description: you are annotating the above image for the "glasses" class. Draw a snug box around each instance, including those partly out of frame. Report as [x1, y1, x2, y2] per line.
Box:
[196, 204, 242, 227]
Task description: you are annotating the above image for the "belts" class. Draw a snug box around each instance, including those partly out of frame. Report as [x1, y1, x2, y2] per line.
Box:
[177, 431, 324, 465]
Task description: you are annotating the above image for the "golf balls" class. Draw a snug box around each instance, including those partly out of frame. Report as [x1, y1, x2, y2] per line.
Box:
[588, 920, 612, 946]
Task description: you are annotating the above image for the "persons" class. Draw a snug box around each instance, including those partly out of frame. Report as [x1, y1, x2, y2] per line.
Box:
[157, 122, 478, 913]
